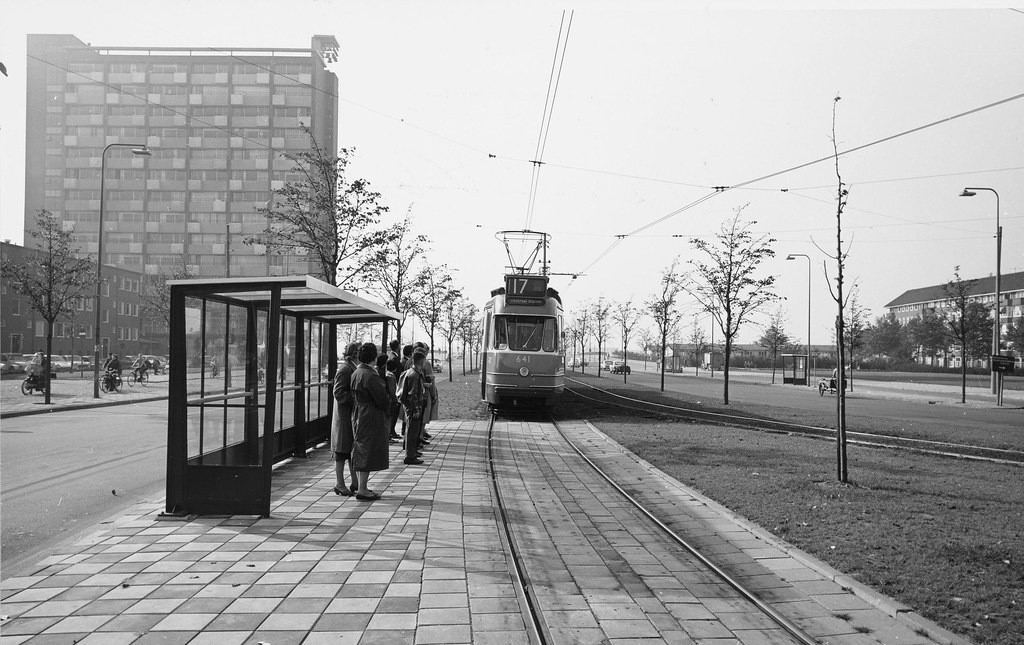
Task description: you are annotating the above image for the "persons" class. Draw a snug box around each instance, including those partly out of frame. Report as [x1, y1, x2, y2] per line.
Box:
[131, 353, 147, 382]
[330, 340, 439, 503]
[211, 353, 221, 376]
[103, 352, 122, 390]
[258, 361, 264, 383]
[145, 356, 161, 375]
[25, 352, 46, 386]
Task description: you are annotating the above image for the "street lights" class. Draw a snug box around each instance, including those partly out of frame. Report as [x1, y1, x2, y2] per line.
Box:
[93, 142, 153, 399]
[958, 187, 1001, 394]
[786, 254, 811, 387]
[697, 286, 714, 377]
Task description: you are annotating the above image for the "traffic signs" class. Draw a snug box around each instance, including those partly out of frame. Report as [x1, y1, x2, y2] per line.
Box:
[991, 354, 1015, 375]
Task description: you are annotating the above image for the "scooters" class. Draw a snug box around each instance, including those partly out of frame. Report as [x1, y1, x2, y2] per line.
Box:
[21, 365, 47, 396]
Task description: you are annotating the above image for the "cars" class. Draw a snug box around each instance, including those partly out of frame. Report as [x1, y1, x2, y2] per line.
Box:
[567, 357, 590, 368]
[0, 352, 29, 375]
[426, 358, 443, 373]
[609, 361, 630, 375]
[22, 351, 170, 374]
[322, 359, 346, 383]
[601, 360, 613, 371]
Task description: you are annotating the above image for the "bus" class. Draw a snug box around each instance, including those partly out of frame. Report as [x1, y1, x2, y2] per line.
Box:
[477, 229, 567, 417]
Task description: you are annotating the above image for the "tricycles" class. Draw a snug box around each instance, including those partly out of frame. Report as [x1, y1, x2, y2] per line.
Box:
[818, 376, 847, 397]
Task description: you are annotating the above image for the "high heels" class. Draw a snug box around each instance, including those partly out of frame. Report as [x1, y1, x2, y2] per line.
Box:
[350, 484, 358, 492]
[334, 487, 353, 495]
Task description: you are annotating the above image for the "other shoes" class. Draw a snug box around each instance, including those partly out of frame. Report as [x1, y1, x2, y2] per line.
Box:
[389, 434, 402, 443]
[402, 431, 432, 458]
[404, 457, 424, 464]
[356, 490, 381, 501]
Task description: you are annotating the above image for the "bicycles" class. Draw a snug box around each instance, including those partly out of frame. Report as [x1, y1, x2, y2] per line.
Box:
[210, 362, 221, 379]
[101, 369, 124, 394]
[126, 365, 150, 388]
[98, 365, 121, 391]
[257, 367, 266, 385]
